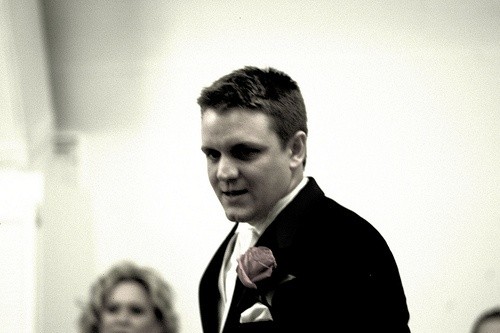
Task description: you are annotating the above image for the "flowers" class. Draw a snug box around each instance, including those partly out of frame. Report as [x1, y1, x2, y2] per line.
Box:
[236, 245, 296, 306]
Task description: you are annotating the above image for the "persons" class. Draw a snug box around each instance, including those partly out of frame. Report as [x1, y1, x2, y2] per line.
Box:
[77, 260, 182, 333]
[197, 65, 411, 333]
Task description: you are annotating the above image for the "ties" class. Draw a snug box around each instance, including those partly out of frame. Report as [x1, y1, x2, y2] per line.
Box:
[222, 227, 255, 318]
